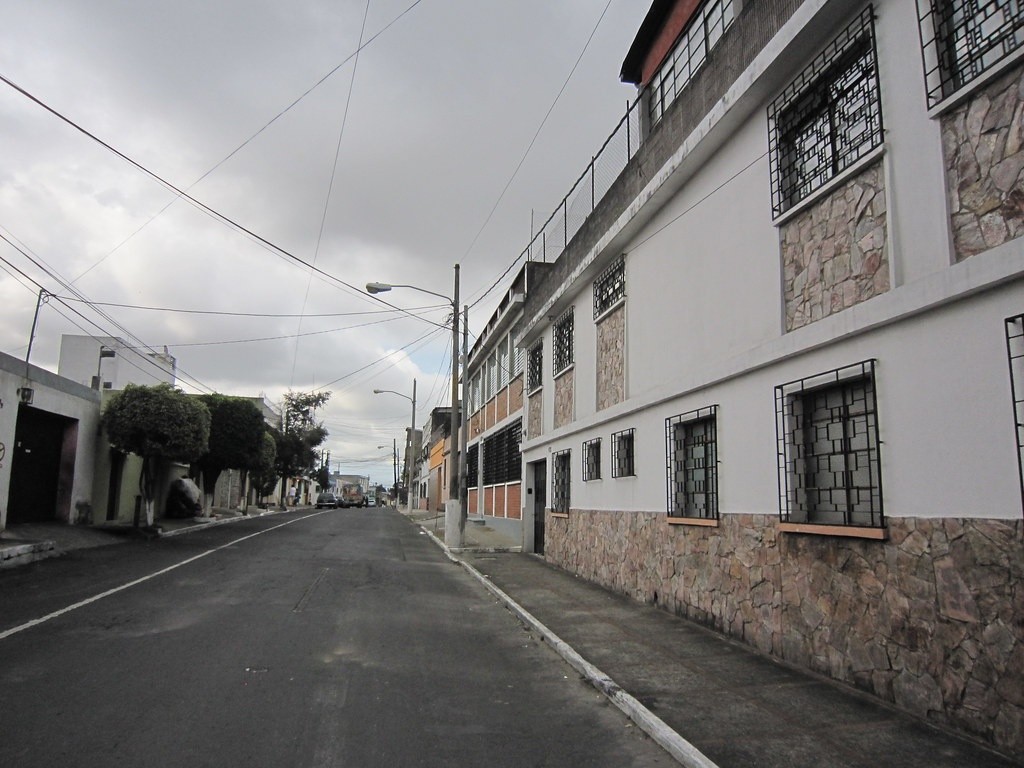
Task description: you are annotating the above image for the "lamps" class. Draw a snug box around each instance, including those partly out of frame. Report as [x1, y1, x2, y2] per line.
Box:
[19, 386, 34, 405]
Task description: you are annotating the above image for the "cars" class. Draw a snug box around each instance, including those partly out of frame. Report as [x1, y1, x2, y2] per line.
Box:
[366, 499, 376, 506]
[315, 492, 339, 509]
[335, 496, 344, 505]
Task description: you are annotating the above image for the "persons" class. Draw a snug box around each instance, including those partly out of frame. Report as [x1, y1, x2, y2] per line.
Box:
[288, 485, 301, 507]
[365, 496, 368, 508]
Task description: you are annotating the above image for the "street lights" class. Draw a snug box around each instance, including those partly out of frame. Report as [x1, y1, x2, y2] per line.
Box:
[373, 377, 417, 514]
[377, 439, 398, 509]
[364, 263, 463, 547]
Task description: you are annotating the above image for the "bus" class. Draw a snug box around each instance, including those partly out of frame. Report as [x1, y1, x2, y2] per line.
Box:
[339, 483, 365, 508]
